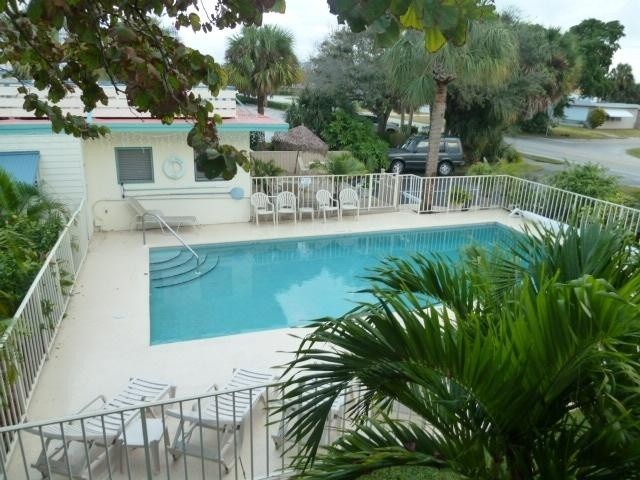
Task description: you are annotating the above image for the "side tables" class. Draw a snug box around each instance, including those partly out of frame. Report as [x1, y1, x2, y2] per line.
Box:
[299, 208, 314, 221]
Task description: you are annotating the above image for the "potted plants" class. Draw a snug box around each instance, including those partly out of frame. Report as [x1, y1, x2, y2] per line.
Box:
[448, 185, 472, 211]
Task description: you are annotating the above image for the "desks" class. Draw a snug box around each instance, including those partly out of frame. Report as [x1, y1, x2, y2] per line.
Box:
[308, 161, 326, 175]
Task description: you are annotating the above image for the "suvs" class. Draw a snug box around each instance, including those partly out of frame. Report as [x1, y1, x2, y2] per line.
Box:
[388, 134, 465, 176]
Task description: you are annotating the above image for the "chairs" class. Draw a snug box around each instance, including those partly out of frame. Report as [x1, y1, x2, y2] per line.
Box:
[250, 191, 298, 227]
[298, 157, 309, 175]
[127, 196, 203, 232]
[263, 174, 282, 194]
[315, 189, 361, 222]
[22, 367, 283, 480]
